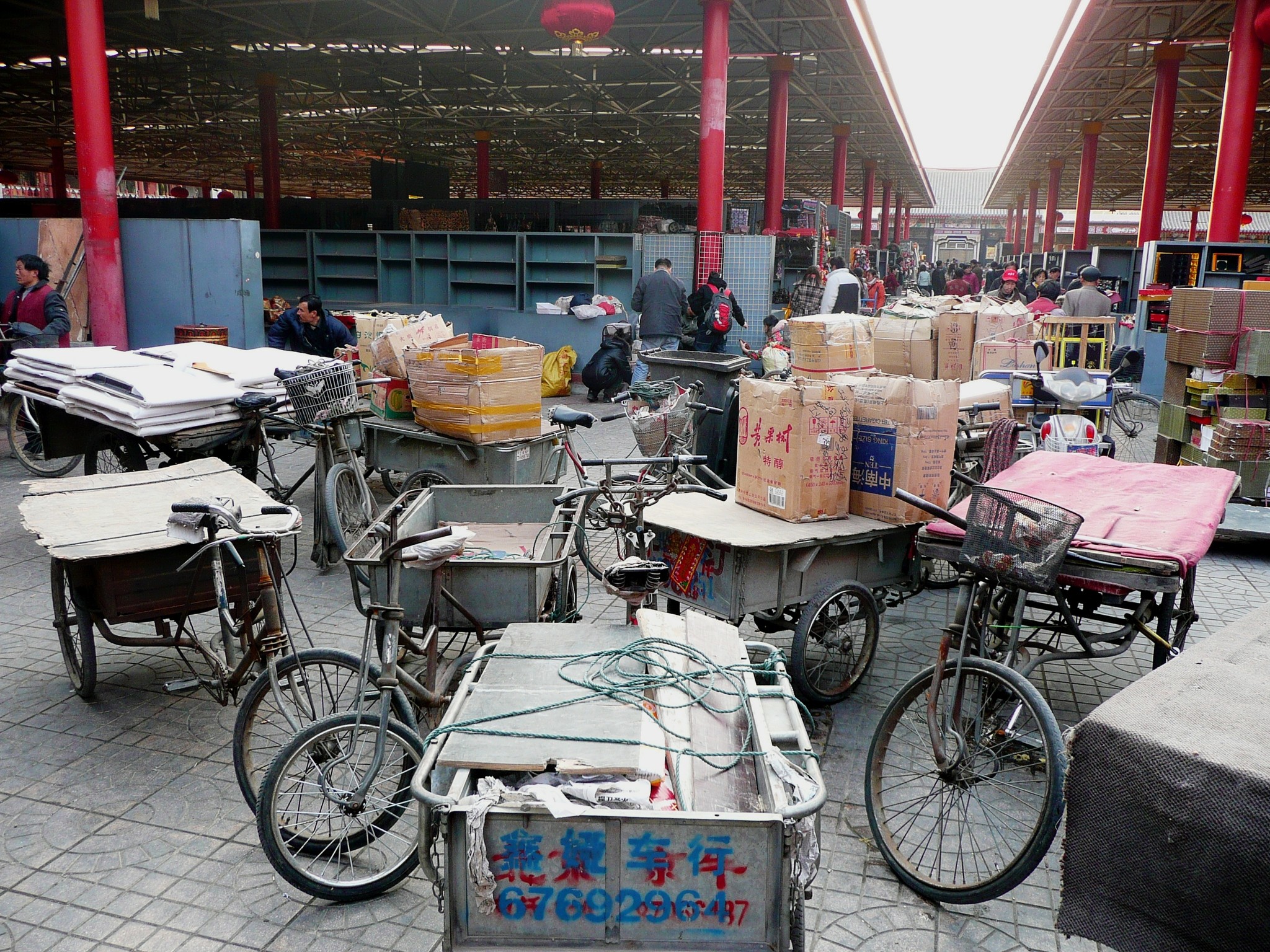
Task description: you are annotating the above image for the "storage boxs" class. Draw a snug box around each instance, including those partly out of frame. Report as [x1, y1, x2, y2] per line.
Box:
[734, 280, 1270, 528]
[333, 308, 544, 444]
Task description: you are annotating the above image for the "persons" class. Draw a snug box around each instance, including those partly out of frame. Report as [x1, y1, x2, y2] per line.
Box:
[739, 314, 792, 376]
[266, 294, 357, 440]
[786, 255, 1112, 371]
[621, 257, 748, 406]
[0, 253, 71, 461]
[580, 327, 633, 403]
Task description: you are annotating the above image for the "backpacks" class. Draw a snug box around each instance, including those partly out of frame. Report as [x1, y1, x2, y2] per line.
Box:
[702, 284, 733, 334]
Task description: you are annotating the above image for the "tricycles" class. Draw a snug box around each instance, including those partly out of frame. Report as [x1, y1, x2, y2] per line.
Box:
[567, 366, 1241, 906]
[0, 319, 655, 903]
[411, 451, 827, 951]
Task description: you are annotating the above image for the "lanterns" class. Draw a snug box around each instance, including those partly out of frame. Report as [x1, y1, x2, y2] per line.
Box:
[1241, 214, 1252, 225]
[1056, 212, 1063, 221]
[858, 210, 864, 219]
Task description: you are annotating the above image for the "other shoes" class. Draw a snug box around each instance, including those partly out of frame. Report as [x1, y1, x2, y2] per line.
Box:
[587, 392, 598, 402]
[603, 395, 611, 402]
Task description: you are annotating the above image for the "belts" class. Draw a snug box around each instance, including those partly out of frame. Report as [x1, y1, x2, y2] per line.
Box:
[1044, 433, 1102, 456]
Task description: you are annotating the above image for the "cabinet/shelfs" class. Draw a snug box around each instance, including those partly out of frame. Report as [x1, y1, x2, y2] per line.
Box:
[120, 217, 643, 374]
[1003, 240, 1270, 400]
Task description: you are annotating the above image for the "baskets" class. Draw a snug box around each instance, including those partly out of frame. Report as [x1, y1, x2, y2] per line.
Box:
[624, 406, 694, 456]
[1105, 350, 1146, 382]
[956, 485, 1084, 592]
[10, 333, 60, 350]
[281, 361, 360, 427]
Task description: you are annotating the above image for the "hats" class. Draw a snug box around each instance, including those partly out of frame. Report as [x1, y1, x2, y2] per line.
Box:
[1080, 267, 1101, 280]
[1002, 269, 1018, 281]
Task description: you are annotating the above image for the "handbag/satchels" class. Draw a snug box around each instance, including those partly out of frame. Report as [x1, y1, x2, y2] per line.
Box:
[781, 302, 792, 319]
